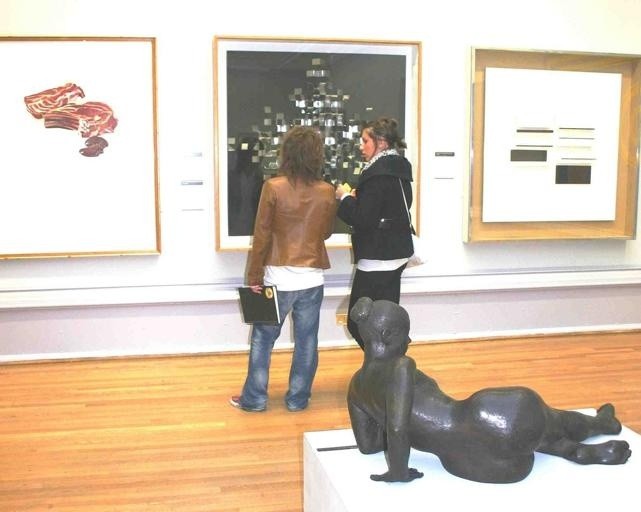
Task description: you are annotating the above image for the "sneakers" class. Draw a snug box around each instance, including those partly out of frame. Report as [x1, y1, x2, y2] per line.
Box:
[229, 394, 242, 408]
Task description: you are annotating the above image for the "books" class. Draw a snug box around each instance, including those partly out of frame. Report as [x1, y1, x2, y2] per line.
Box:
[235, 283, 281, 325]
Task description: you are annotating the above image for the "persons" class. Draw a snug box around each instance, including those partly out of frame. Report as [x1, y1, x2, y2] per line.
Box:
[346, 295, 633, 484]
[334, 116, 415, 352]
[228, 123, 337, 413]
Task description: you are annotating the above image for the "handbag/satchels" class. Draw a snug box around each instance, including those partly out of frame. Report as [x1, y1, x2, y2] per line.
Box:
[406, 232, 432, 271]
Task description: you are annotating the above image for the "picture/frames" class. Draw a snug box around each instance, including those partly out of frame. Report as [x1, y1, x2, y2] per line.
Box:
[214, 35, 424, 254]
[465, 44, 640, 244]
[0, 35, 163, 261]
